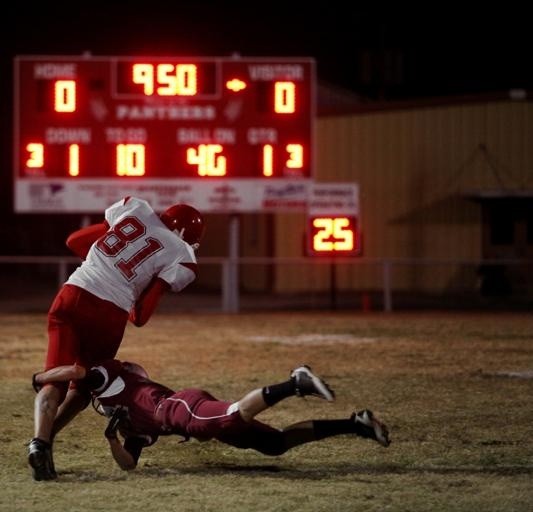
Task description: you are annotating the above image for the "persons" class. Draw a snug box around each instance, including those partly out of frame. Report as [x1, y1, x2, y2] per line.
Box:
[27, 195, 206, 482]
[31, 361, 391, 471]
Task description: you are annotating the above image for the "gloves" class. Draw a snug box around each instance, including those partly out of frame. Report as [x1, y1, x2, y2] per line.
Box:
[33, 372, 42, 391]
[104, 410, 124, 438]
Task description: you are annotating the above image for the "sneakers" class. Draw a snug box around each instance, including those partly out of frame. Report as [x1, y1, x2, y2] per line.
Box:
[291, 365, 335, 403]
[351, 408, 391, 448]
[27, 438, 58, 481]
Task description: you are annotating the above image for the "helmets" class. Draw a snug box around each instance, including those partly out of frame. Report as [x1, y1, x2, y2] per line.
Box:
[160, 204, 204, 249]
